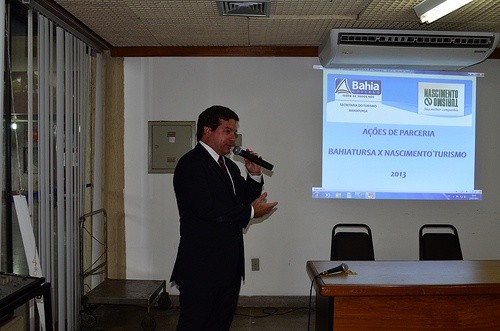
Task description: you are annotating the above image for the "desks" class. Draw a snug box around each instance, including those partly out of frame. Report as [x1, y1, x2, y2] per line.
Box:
[306, 260, 500, 331]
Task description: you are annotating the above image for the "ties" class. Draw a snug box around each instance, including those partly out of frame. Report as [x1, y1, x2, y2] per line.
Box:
[218, 156, 225, 168]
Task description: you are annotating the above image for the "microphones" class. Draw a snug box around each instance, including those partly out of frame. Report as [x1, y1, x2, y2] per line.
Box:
[321, 263, 348, 275]
[233, 146, 273, 171]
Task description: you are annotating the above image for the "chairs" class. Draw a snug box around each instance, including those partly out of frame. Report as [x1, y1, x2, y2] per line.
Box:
[331, 224, 375, 261]
[80, 208, 167, 331]
[419, 224, 463, 261]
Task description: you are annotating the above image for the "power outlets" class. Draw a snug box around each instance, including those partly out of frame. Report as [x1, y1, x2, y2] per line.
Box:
[252, 258, 259, 271]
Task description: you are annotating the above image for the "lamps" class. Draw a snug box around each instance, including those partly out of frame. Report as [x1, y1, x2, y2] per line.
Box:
[413, 0, 475, 24]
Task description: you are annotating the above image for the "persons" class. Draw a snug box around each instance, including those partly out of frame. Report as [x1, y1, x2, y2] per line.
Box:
[168, 105, 278, 331]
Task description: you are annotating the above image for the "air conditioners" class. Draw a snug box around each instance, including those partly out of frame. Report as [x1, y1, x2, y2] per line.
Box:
[318, 28, 500, 71]
